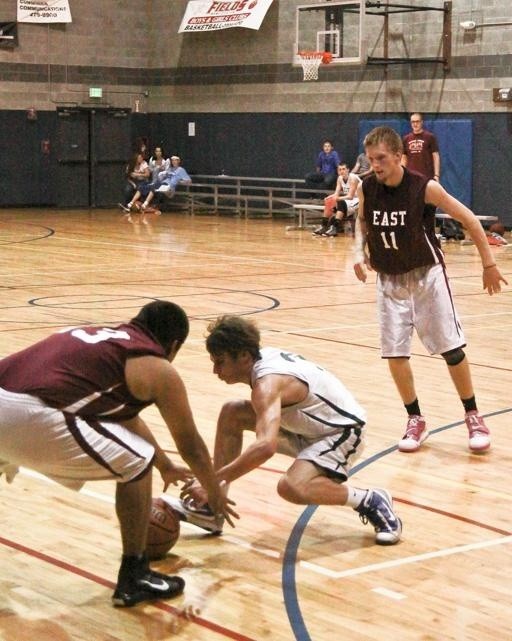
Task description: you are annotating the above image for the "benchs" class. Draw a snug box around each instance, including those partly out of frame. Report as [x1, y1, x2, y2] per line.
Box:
[286, 204, 499, 246]
[163, 173, 305, 220]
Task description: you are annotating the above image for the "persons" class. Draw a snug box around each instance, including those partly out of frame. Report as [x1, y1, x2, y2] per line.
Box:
[147, 146, 166, 185]
[162, 316, 401, 546]
[351, 139, 374, 181]
[126, 152, 150, 204]
[301, 140, 341, 200]
[399, 113, 441, 240]
[118, 155, 192, 214]
[350, 124, 508, 454]
[1, 300, 241, 607]
[311, 162, 362, 237]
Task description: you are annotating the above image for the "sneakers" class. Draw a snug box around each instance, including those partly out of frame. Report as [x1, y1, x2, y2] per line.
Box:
[322, 226, 338, 237]
[312, 227, 328, 235]
[399, 413, 431, 454]
[131, 204, 144, 213]
[111, 568, 187, 609]
[465, 409, 494, 456]
[160, 496, 227, 535]
[118, 201, 131, 212]
[359, 488, 406, 545]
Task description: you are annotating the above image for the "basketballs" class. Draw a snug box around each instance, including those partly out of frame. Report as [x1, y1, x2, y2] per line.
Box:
[131, 201, 144, 213]
[149, 498, 179, 559]
[490, 222, 505, 235]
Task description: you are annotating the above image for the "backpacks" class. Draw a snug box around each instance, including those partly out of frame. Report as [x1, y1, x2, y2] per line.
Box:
[440, 218, 466, 240]
